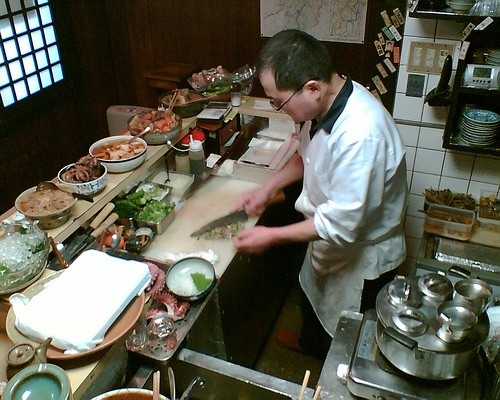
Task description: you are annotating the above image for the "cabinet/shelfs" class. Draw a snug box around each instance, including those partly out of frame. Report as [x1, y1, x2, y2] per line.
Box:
[442, 22, 500, 156]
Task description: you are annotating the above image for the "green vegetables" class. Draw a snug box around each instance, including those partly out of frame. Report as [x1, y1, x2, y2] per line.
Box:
[190, 273, 212, 292]
[0, 226, 45, 274]
[203, 78, 233, 95]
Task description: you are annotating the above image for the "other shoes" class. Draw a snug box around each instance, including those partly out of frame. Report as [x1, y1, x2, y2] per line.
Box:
[274, 329, 305, 353]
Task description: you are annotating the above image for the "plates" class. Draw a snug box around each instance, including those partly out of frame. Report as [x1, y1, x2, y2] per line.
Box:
[90, 388, 170, 400]
[458, 109, 500, 145]
[5, 268, 145, 359]
[3, 364, 74, 400]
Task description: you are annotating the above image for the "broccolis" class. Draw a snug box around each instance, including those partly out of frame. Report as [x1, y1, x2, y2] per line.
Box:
[116, 189, 175, 223]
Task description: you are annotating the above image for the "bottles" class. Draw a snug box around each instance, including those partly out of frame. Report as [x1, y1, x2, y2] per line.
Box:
[174, 145, 190, 175]
[188, 135, 205, 176]
[230, 83, 242, 106]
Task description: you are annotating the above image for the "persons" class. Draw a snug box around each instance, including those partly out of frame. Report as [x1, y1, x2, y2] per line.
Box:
[229, 30, 408, 355]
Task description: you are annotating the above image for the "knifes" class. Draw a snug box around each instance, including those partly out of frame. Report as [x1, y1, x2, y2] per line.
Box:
[62, 203, 119, 262]
[189, 189, 286, 237]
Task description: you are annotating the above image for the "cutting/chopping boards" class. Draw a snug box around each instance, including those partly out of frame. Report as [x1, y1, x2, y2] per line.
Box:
[140, 175, 270, 281]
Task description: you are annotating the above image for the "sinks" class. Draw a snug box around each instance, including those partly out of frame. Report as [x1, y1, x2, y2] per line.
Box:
[123, 348, 314, 400]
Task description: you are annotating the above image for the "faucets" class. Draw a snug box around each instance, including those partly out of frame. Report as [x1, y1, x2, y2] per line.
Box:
[179, 375, 206, 400]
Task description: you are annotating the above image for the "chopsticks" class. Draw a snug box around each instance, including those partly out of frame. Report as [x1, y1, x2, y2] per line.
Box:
[153, 371, 160, 400]
[184, 96, 215, 104]
[167, 92, 180, 110]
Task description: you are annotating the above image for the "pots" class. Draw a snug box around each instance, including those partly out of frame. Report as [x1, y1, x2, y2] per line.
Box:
[375, 274, 490, 380]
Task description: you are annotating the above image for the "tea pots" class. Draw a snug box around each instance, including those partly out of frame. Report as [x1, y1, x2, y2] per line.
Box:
[6, 337, 53, 381]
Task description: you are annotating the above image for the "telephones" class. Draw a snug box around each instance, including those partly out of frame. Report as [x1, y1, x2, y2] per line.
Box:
[424, 55, 452, 106]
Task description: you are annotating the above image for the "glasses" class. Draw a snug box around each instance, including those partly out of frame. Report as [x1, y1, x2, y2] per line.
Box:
[270, 77, 320, 112]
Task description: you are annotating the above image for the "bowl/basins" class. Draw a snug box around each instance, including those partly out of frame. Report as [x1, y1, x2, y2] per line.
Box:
[16, 184, 78, 230]
[175, 88, 230, 102]
[423, 193, 500, 240]
[117, 202, 176, 236]
[146, 168, 195, 197]
[165, 258, 215, 301]
[88, 135, 147, 173]
[0, 224, 49, 290]
[159, 90, 204, 118]
[128, 110, 182, 144]
[58, 163, 107, 196]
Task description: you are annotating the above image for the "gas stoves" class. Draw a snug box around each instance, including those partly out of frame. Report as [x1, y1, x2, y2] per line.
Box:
[337, 309, 465, 400]
